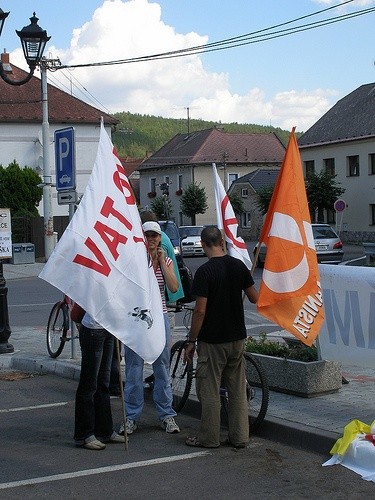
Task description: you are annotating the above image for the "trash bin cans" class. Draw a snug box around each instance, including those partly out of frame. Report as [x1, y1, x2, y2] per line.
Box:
[12, 242, 35, 264]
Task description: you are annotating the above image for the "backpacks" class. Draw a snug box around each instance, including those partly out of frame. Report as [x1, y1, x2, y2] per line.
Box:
[69, 303, 85, 323]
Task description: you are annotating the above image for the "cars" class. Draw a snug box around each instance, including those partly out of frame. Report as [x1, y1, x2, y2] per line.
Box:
[179, 225, 208, 257]
[252, 224, 344, 266]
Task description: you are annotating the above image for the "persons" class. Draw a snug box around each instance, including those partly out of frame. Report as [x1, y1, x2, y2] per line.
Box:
[74, 312, 129, 450]
[120, 221, 180, 435]
[186, 226, 259, 448]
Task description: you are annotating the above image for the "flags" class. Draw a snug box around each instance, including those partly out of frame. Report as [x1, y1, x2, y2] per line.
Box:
[38, 120, 165, 365]
[256, 135, 325, 346]
[214, 168, 253, 271]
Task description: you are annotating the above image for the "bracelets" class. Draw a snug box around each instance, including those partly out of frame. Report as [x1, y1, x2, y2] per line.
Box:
[189, 339, 196, 343]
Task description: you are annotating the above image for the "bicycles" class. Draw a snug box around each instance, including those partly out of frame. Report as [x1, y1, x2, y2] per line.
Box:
[45, 300, 76, 359]
[154, 305, 270, 433]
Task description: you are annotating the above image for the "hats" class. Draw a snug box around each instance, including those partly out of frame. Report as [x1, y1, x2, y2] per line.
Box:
[141, 221, 161, 235]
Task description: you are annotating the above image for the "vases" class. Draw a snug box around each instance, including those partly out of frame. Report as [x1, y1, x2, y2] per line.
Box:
[245, 352, 344, 399]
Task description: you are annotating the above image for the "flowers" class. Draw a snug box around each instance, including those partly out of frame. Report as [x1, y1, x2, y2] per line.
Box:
[245, 334, 319, 363]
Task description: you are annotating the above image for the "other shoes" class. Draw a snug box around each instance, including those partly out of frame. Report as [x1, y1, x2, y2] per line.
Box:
[96, 430, 129, 443]
[225, 438, 244, 448]
[185, 433, 202, 446]
[76, 437, 106, 450]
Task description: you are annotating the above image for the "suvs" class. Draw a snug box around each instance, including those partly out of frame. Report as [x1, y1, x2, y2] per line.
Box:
[158, 220, 188, 268]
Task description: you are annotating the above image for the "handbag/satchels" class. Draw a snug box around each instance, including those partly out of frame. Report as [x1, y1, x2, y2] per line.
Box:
[177, 265, 197, 303]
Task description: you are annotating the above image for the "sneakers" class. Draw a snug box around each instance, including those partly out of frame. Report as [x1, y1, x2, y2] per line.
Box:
[159, 415, 180, 433]
[118, 418, 136, 434]
